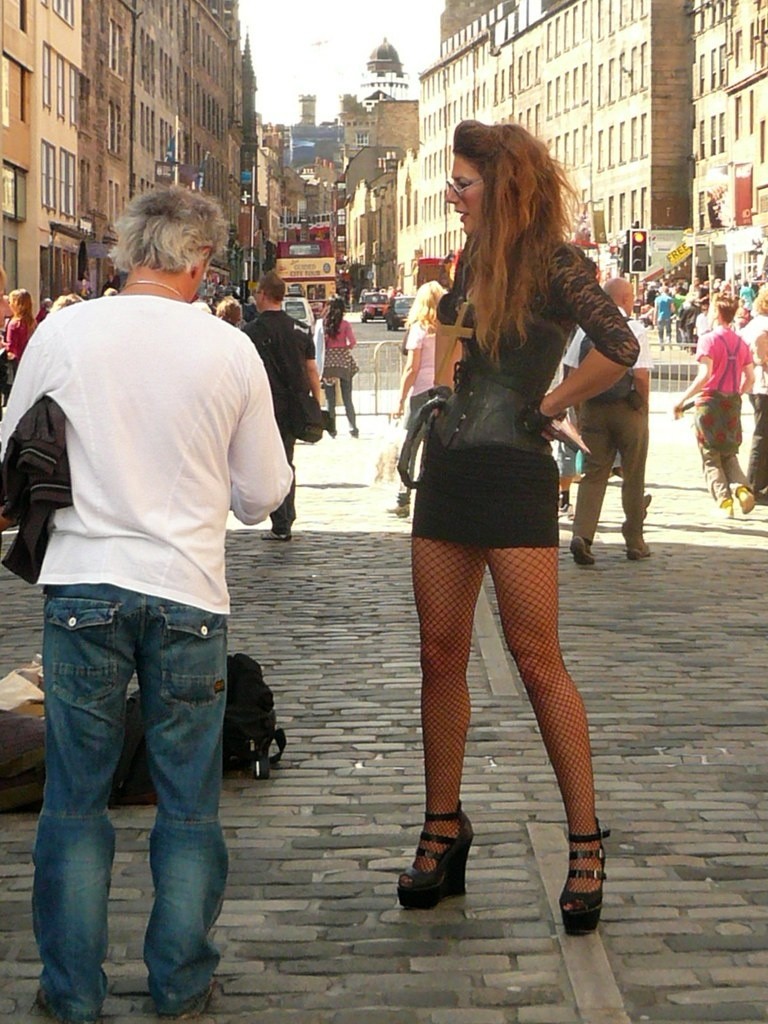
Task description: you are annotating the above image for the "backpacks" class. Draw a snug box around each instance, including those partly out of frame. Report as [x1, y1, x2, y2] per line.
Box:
[578, 317, 635, 405]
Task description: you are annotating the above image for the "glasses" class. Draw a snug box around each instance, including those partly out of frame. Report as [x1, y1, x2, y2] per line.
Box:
[446, 177, 486, 198]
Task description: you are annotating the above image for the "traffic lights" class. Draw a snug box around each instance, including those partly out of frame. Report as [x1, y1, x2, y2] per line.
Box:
[629, 229, 649, 273]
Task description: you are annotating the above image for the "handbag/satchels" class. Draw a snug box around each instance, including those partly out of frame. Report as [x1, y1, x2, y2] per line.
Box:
[291, 392, 323, 443]
[109, 652, 287, 805]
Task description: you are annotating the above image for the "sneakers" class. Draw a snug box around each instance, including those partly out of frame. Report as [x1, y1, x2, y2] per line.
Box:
[557, 504, 574, 520]
[641, 494, 651, 519]
[260, 530, 291, 541]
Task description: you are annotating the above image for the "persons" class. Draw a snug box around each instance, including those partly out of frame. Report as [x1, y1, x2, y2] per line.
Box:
[390, 272, 768, 565]
[0, 182, 295, 1024]
[396, 121, 641, 936]
[0, 270, 359, 540]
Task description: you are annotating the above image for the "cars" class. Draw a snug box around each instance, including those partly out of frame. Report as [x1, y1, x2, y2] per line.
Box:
[281, 298, 315, 335]
[361, 293, 390, 323]
[385, 296, 416, 331]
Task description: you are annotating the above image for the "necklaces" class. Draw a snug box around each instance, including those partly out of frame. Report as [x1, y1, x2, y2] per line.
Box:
[122, 280, 186, 299]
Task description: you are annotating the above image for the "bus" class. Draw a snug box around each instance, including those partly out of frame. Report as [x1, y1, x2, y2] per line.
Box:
[276, 240, 337, 321]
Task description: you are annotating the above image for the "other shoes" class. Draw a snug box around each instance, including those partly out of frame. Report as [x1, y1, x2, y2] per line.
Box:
[37, 983, 53, 1014]
[625, 544, 650, 559]
[755, 486, 768, 504]
[719, 499, 734, 519]
[737, 488, 755, 513]
[385, 504, 411, 517]
[158, 977, 215, 1024]
[330, 431, 336, 437]
[348, 428, 359, 437]
[570, 536, 595, 564]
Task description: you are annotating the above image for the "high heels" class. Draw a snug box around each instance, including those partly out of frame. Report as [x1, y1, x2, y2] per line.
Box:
[398, 798, 473, 908]
[559, 818, 610, 934]
